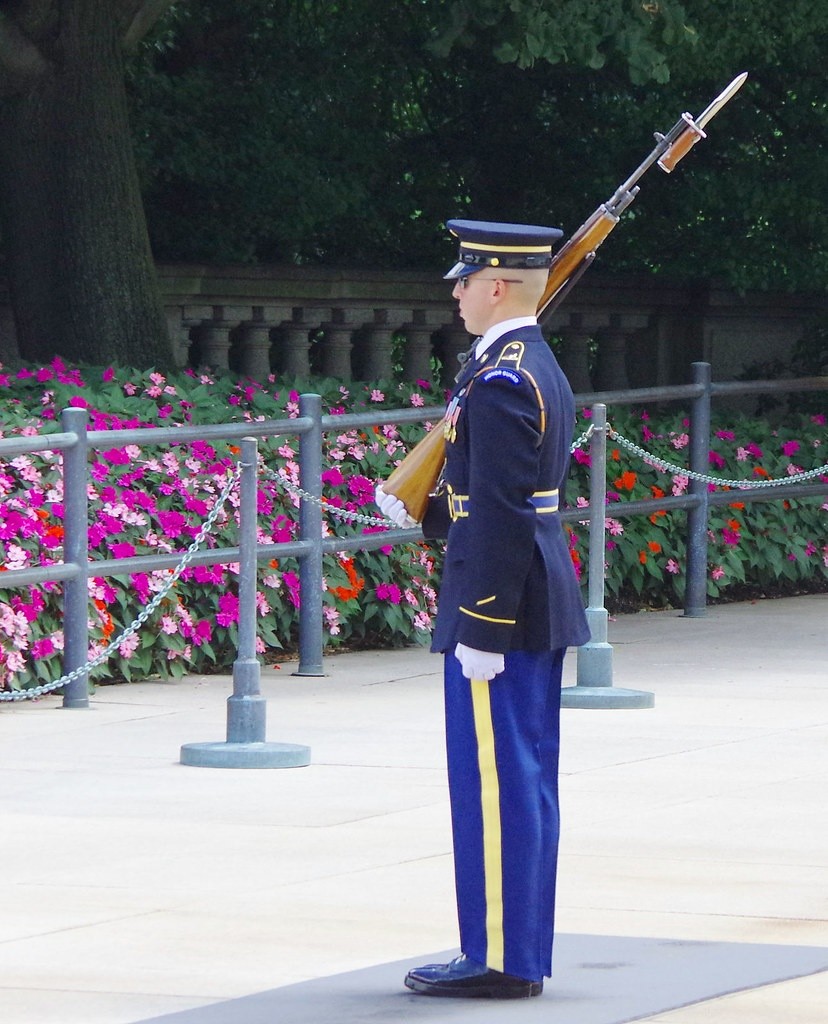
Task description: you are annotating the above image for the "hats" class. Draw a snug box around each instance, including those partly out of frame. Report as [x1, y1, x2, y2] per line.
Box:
[443, 220, 564, 279]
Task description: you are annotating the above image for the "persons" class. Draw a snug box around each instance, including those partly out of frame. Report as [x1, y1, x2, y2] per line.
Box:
[375, 218, 593, 999]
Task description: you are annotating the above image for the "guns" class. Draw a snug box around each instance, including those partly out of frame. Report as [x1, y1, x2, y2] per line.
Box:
[377, 71, 750, 526]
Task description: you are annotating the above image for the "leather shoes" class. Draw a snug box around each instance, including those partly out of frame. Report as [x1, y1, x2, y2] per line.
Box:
[405, 953, 543, 999]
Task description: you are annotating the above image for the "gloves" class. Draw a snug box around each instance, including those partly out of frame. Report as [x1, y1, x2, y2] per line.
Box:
[376, 485, 421, 528]
[454, 643, 505, 680]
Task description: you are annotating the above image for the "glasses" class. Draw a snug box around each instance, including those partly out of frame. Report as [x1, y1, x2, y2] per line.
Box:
[459, 276, 524, 288]
